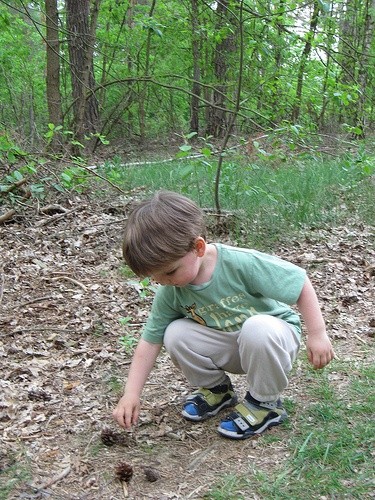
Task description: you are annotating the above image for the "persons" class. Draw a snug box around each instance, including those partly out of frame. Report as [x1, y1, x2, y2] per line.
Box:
[111, 190, 335, 438]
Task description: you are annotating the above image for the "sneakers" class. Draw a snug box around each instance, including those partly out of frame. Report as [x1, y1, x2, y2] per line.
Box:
[182, 375, 237, 421]
[217, 391, 288, 440]
